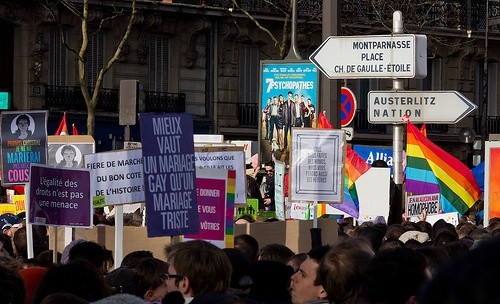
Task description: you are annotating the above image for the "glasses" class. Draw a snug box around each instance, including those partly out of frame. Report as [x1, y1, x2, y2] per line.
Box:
[266, 170, 274, 173]
[162, 273, 179, 280]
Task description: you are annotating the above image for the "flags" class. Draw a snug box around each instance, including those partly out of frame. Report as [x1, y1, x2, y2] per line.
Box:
[317, 113, 372, 219]
[406, 119, 483, 216]
[53, 113, 78, 136]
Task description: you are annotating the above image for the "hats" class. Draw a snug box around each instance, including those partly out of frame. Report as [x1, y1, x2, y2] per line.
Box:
[0, 213, 22, 231]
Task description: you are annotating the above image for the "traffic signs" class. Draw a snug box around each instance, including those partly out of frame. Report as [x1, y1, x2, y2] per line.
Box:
[367, 89, 479, 124]
[308, 34, 417, 80]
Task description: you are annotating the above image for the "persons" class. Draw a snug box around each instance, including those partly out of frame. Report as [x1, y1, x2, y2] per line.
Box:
[246, 176, 263, 212]
[0, 199, 500, 304]
[263, 160, 276, 211]
[264, 90, 315, 150]
[370, 160, 401, 226]
